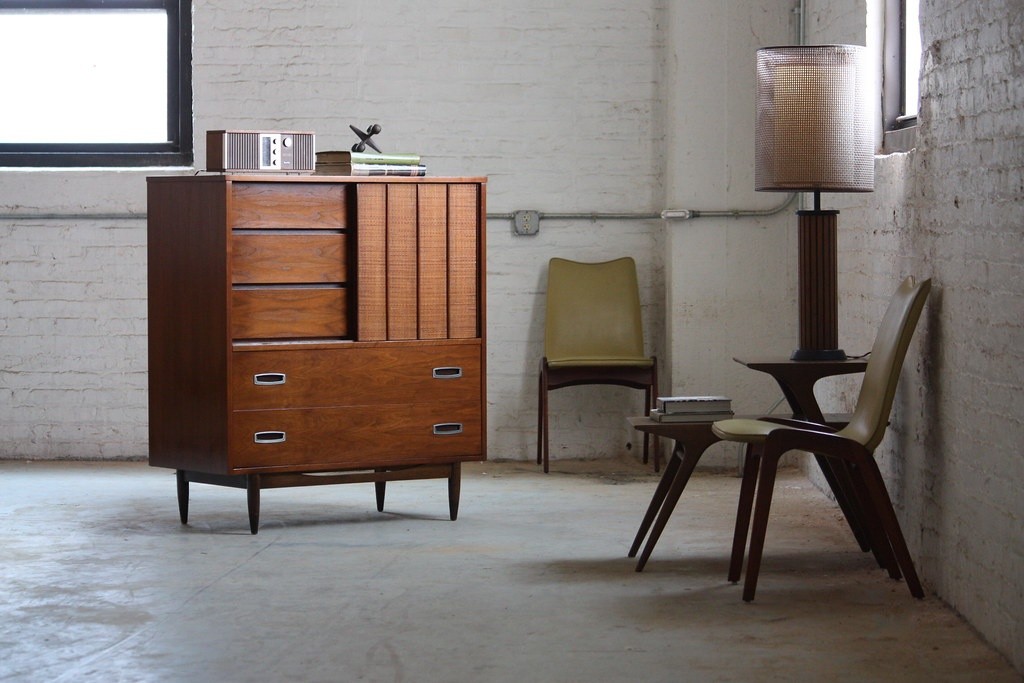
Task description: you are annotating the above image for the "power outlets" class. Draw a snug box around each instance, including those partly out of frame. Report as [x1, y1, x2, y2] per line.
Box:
[512, 210, 540, 235]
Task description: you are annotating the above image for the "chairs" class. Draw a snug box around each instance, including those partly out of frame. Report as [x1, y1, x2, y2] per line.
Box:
[537, 257, 660, 474]
[711, 276, 932, 605]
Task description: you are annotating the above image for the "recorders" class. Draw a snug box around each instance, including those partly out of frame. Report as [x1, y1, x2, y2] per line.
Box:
[207, 130, 317, 176]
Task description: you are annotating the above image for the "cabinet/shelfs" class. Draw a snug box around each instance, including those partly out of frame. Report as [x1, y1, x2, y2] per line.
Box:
[145, 174, 488, 534]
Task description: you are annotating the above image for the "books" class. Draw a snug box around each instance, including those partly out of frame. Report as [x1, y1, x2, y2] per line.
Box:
[310, 164, 426, 177]
[656, 396, 734, 413]
[650, 409, 733, 424]
[315, 151, 420, 165]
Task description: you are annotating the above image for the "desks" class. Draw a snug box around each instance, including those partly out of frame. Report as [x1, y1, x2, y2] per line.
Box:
[625, 355, 870, 572]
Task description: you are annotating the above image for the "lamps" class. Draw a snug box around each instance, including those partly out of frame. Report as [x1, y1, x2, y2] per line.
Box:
[754, 44, 872, 361]
